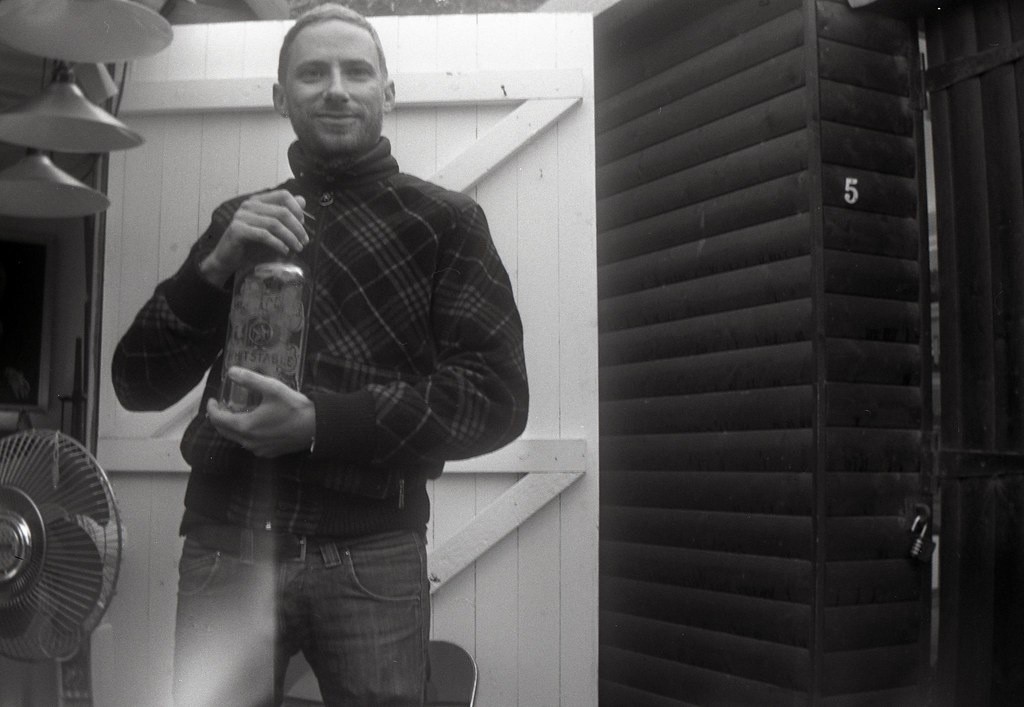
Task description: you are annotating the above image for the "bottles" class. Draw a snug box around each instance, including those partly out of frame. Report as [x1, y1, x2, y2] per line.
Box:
[220, 247, 315, 414]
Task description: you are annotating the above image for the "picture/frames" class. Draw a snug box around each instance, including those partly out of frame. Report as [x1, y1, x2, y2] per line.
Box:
[0, 227, 58, 417]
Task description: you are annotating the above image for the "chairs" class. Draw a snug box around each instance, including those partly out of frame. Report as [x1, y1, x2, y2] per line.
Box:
[423, 639, 478, 707]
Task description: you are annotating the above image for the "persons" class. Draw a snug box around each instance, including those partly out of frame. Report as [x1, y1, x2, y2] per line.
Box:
[111, 4, 530, 707]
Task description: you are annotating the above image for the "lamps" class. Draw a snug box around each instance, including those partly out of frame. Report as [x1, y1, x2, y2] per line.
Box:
[0, 0, 174, 218]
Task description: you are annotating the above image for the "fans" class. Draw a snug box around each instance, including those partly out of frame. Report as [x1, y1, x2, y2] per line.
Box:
[0, 428, 123, 707]
